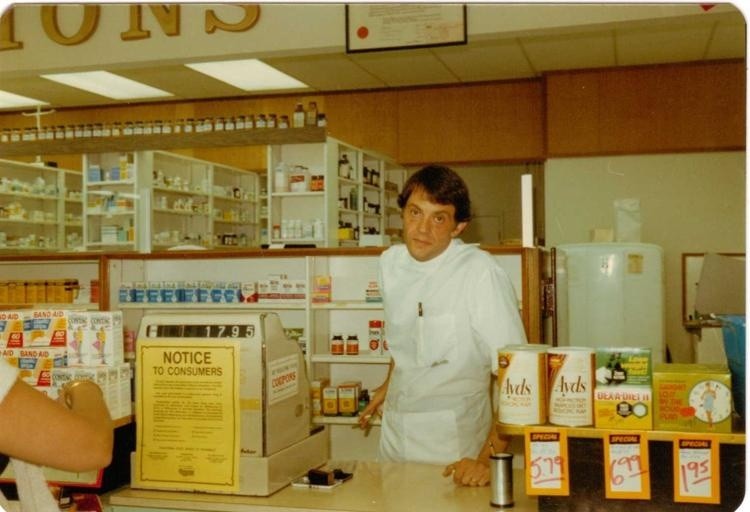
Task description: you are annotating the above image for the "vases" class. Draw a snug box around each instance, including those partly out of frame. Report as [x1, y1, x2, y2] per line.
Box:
[344, 2, 469, 52]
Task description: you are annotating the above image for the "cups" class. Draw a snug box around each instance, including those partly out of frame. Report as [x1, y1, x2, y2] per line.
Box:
[488, 451, 513, 506]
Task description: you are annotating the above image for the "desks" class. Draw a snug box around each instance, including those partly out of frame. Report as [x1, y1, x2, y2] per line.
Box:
[500, 423, 745, 510]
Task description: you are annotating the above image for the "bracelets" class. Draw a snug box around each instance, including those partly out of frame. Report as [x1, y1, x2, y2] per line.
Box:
[65, 380, 104, 408]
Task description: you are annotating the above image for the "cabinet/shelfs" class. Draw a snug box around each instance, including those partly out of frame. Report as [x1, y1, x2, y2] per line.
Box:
[323, 138, 407, 249]
[0, 255, 99, 313]
[265, 136, 338, 249]
[83, 153, 139, 254]
[0, 159, 84, 255]
[99, 250, 304, 409]
[131, 149, 261, 249]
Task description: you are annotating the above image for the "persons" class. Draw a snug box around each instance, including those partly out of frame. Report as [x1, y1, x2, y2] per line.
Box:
[1, 351, 116, 512]
[353, 165, 530, 490]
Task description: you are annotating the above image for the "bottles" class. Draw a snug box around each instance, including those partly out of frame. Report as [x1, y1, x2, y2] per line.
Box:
[273, 160, 311, 192]
[358, 389, 369, 413]
[272, 217, 324, 242]
[339, 156, 358, 240]
[363, 167, 381, 235]
[1, 100, 327, 141]
[347, 332, 359, 354]
[328, 330, 345, 356]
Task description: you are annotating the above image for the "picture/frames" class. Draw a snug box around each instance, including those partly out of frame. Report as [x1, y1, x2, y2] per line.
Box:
[680, 247, 746, 329]
[304, 250, 542, 424]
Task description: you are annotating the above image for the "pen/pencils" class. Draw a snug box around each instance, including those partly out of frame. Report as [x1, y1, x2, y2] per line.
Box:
[488, 440, 496, 457]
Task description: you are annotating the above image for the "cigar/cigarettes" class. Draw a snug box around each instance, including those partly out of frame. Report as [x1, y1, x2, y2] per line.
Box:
[360, 414, 371, 422]
